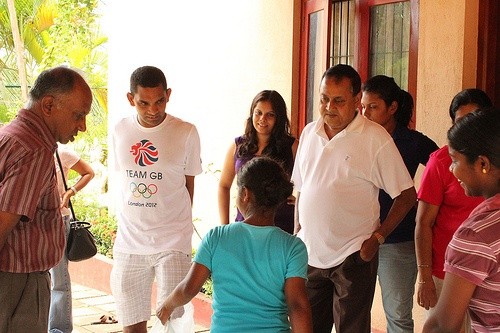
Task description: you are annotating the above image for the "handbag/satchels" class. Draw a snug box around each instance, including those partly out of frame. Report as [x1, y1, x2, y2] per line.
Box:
[66, 218, 97, 261]
[147, 316, 175, 333]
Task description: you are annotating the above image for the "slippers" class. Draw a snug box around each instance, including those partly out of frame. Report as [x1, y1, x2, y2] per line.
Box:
[93, 314, 118, 324]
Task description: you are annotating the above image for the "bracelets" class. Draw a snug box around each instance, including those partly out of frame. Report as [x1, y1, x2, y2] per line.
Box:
[374, 231, 385, 245]
[416, 279, 433, 284]
[418, 264, 433, 268]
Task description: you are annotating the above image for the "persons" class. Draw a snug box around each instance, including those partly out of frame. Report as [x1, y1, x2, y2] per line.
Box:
[110, 66, 200, 333]
[211, 90, 309, 254]
[157, 155, 322, 333]
[347, 74, 444, 333]
[1, 66, 106, 333]
[413, 87, 500, 333]
[282, 65, 419, 333]
